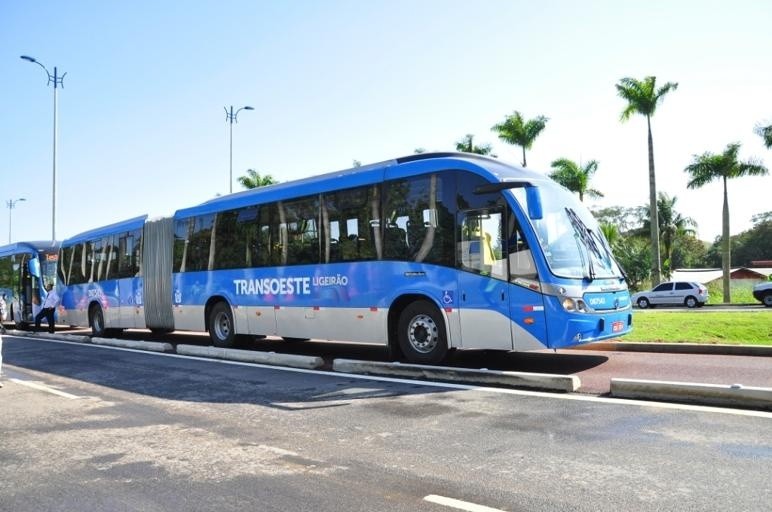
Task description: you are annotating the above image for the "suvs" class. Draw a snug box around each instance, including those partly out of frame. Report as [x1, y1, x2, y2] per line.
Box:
[630, 279, 711, 308]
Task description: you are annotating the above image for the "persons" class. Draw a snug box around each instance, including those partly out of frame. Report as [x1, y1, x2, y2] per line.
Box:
[32, 278, 59, 333]
[0, 293, 7, 324]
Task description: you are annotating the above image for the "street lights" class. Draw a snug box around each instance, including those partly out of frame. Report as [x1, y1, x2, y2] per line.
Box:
[9, 197, 27, 244]
[222, 104, 258, 197]
[18, 51, 69, 246]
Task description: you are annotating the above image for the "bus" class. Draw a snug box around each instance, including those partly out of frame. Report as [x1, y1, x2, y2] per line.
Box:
[52, 144, 636, 372]
[0, 238, 80, 331]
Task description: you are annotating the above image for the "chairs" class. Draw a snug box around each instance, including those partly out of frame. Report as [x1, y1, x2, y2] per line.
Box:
[228, 221, 456, 269]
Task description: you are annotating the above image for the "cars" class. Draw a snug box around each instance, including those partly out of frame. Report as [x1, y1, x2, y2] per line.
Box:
[752, 280, 772, 308]
